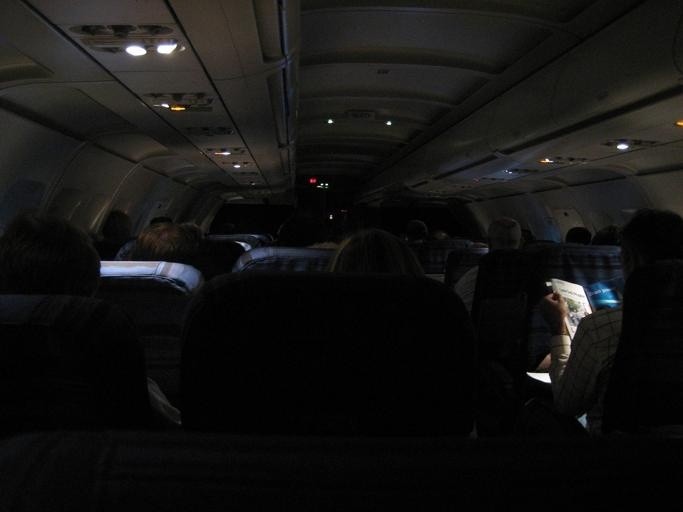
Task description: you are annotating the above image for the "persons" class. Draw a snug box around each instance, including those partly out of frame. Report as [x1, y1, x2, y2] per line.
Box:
[411, 216, 623, 312]
[55, 213, 239, 263]
[2, 214, 180, 428]
[312, 228, 423, 276]
[540, 214, 681, 428]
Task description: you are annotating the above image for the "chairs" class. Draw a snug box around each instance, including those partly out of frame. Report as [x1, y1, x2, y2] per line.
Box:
[0, 235, 682, 512]
